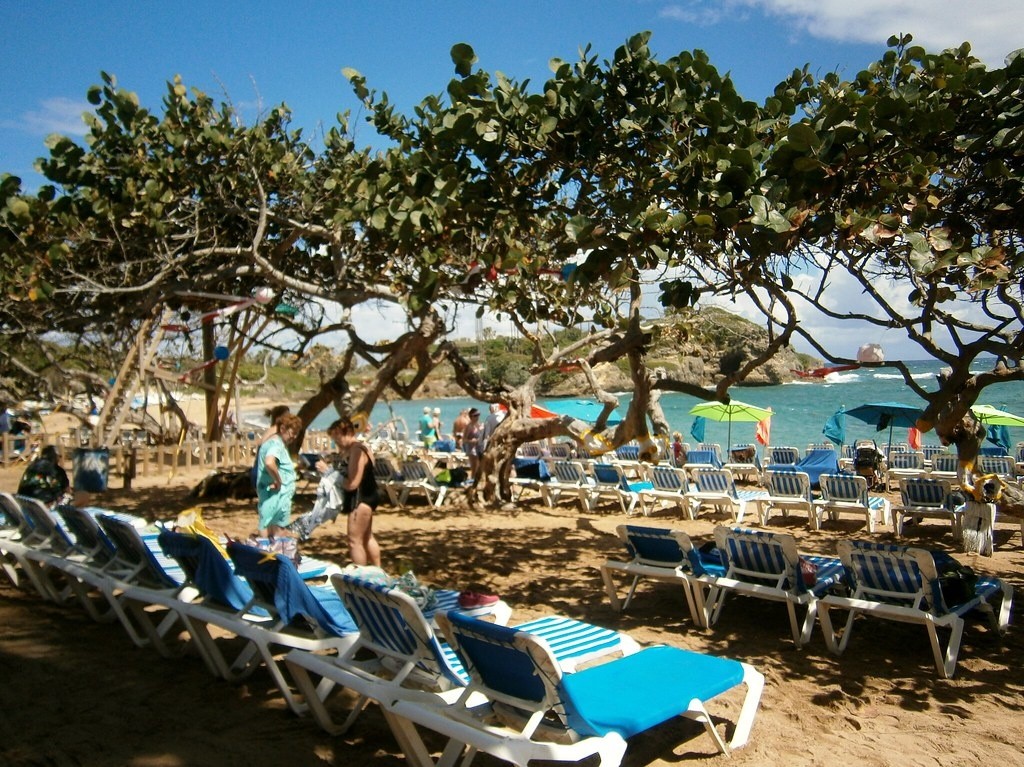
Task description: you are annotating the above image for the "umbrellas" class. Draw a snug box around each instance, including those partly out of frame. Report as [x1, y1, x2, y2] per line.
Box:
[490, 401, 560, 423]
[842, 401, 925, 470]
[822, 404, 846, 446]
[970, 404, 1024, 426]
[756, 407, 772, 445]
[986, 405, 1012, 449]
[907, 427, 921, 451]
[543, 399, 624, 424]
[690, 416, 705, 443]
[688, 398, 776, 459]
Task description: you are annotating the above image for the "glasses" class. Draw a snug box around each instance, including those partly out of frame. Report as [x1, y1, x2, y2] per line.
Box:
[471, 413, 480, 417]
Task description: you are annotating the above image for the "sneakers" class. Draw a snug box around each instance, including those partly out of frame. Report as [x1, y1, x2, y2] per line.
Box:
[458, 583, 498, 609]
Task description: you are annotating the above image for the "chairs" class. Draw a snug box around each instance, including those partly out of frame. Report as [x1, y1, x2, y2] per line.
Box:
[0, 439, 1024, 767]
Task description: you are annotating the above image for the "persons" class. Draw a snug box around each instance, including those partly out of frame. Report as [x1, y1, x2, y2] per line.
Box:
[0, 401, 31, 464]
[256, 406, 302, 536]
[18, 445, 69, 503]
[315, 415, 380, 567]
[673, 431, 688, 468]
[418, 403, 505, 449]
[462, 408, 485, 480]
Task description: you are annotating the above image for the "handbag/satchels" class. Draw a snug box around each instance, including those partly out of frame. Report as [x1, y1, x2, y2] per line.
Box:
[154, 507, 230, 560]
[394, 570, 439, 613]
[928, 549, 976, 609]
[246, 533, 299, 571]
[433, 466, 468, 486]
[699, 541, 723, 567]
[798, 556, 818, 586]
